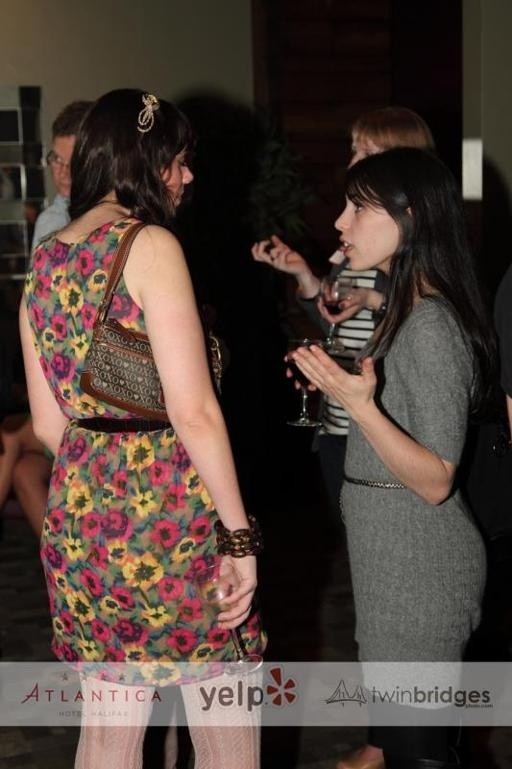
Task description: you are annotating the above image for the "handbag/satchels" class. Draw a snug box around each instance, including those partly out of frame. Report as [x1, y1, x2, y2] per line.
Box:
[80, 222, 224, 422]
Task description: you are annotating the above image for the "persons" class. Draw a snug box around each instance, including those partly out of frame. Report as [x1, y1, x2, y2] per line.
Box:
[2, 88, 512, 768]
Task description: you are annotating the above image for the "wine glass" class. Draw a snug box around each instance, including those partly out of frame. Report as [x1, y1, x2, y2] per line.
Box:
[193, 560, 263, 674]
[315, 275, 355, 353]
[284, 336, 326, 428]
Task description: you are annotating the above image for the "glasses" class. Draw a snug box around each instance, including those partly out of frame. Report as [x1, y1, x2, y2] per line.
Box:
[46, 150, 71, 170]
